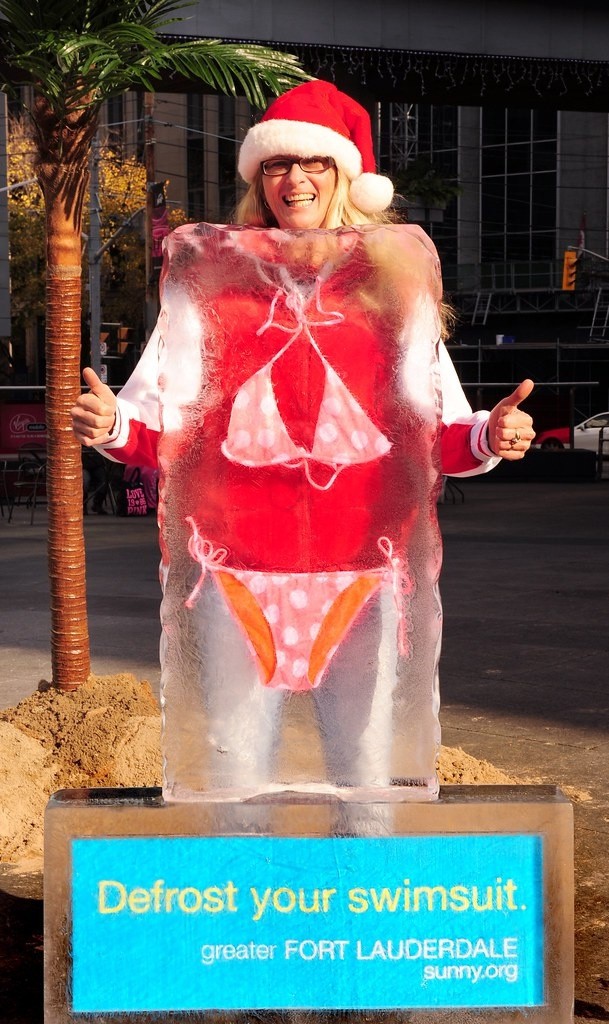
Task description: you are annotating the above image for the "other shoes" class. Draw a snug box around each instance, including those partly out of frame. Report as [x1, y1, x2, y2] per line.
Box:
[92, 503, 108, 515]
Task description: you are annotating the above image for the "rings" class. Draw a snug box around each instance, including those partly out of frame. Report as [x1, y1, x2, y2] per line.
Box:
[510, 437, 517, 448]
[514, 428, 520, 440]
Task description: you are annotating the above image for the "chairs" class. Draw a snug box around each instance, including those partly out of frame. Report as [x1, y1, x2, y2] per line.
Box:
[0, 442, 117, 526]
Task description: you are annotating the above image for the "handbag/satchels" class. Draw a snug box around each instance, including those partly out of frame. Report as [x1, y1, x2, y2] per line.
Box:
[116, 466, 148, 517]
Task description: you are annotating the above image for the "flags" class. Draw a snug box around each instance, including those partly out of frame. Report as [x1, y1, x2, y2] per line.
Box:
[576, 211, 588, 275]
[150, 182, 171, 259]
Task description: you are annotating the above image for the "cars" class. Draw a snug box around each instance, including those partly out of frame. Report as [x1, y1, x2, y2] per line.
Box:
[533, 410, 609, 455]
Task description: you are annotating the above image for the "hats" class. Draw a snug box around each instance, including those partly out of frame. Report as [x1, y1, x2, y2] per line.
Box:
[238, 80, 393, 214]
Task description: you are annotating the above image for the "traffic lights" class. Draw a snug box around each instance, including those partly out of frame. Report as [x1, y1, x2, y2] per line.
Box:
[118, 328, 135, 354]
[562, 251, 577, 290]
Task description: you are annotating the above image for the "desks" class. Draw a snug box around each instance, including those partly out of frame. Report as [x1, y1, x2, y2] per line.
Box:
[0, 454, 48, 518]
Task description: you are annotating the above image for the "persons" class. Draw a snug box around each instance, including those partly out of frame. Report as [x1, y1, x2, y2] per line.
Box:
[82, 442, 109, 516]
[63, 82, 538, 837]
[0, 336, 13, 386]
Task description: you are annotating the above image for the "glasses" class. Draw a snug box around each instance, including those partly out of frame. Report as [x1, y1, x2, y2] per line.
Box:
[260, 155, 336, 176]
